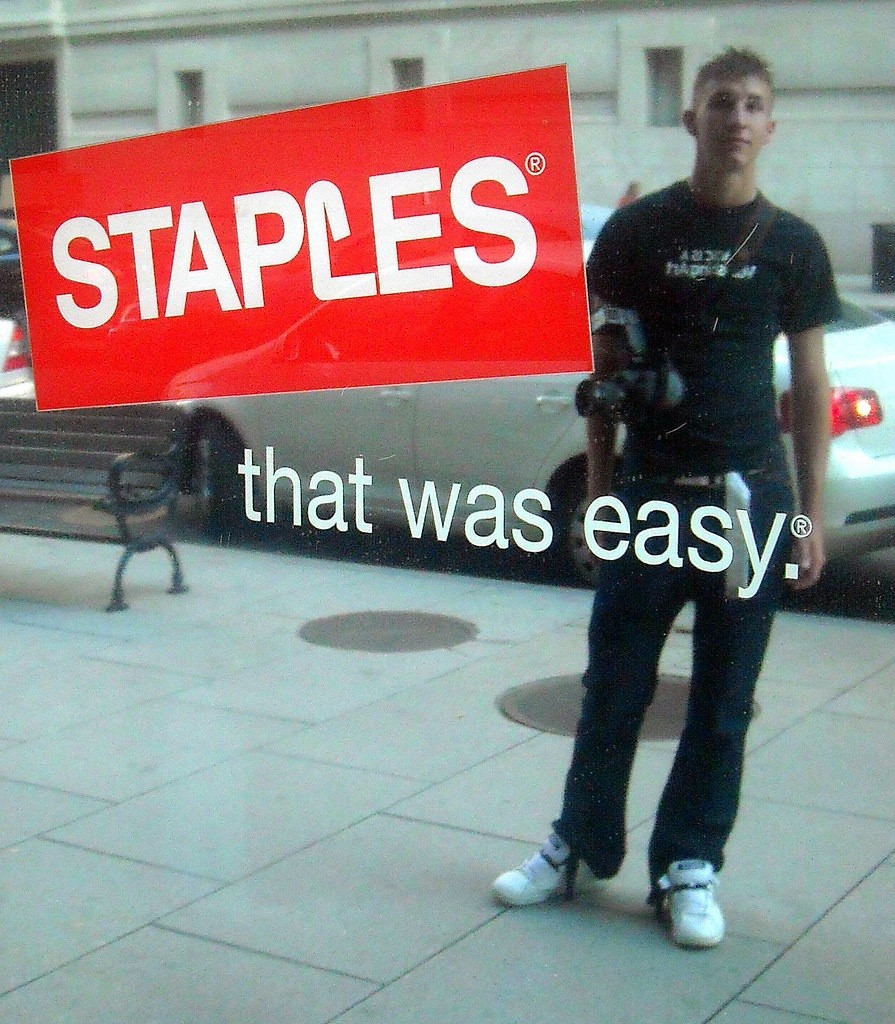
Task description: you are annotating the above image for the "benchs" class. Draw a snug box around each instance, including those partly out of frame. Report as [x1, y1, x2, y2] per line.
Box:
[0, 396, 188, 613]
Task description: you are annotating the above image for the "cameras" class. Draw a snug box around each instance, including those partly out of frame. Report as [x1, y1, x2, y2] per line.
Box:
[576, 306, 685, 421]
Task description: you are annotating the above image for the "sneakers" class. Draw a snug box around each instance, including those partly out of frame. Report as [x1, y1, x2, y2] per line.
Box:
[492, 829, 599, 907]
[657, 857, 725, 949]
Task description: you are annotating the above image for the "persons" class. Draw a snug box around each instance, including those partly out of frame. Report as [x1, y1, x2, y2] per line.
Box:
[491, 46, 844, 946]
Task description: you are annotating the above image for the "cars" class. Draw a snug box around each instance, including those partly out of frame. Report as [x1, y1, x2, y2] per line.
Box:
[180, 237, 893, 591]
[0, 314, 34, 394]
